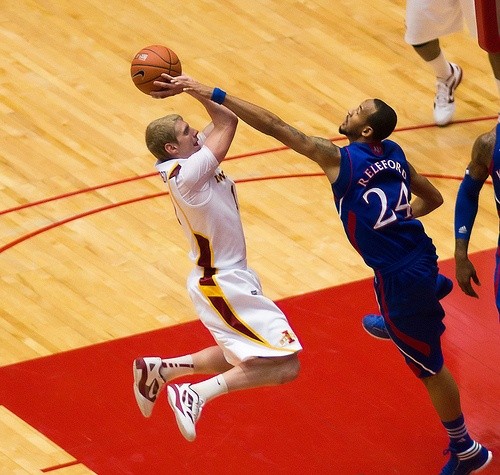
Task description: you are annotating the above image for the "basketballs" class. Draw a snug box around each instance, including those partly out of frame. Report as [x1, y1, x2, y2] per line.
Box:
[131, 45, 182, 95]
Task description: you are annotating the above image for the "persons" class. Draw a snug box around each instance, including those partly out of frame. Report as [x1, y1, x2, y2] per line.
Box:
[453, 120, 500, 322]
[131, 72, 305, 442]
[171, 74, 494, 475]
[404, 0, 500, 127]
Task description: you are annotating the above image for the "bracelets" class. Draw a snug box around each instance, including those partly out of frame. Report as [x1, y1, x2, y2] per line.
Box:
[211, 88, 226, 105]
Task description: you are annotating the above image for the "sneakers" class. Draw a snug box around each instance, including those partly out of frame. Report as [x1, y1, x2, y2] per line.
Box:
[440, 440, 493, 475]
[167, 382, 204, 442]
[133, 357, 165, 418]
[433, 62, 462, 126]
[362, 314, 392, 340]
